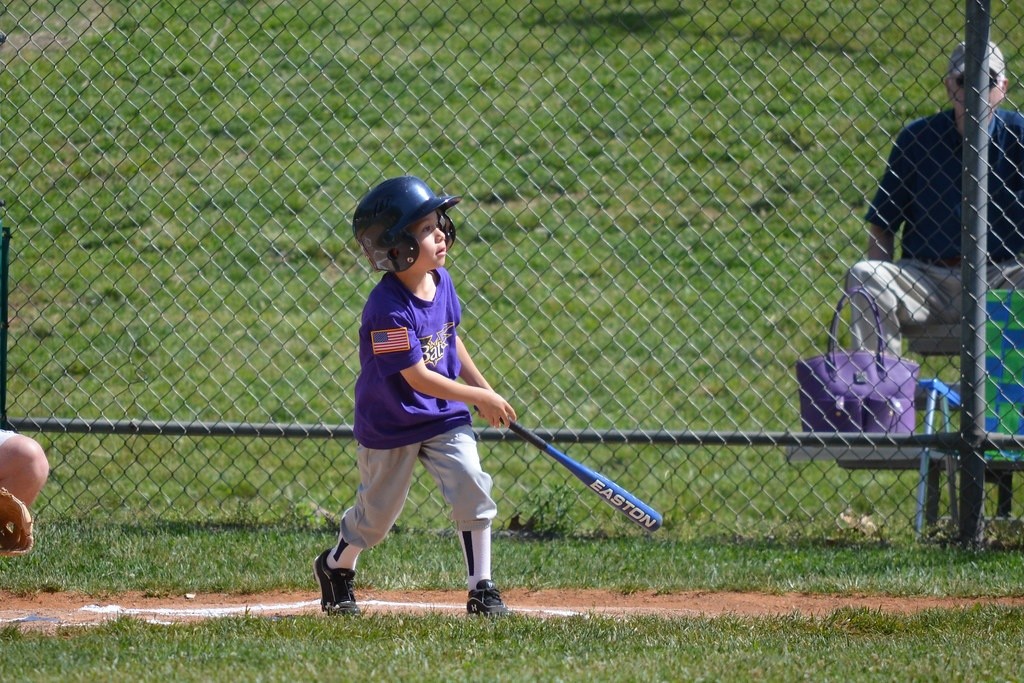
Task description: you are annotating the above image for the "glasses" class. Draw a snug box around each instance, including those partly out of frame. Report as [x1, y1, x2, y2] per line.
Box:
[951, 73, 999, 90]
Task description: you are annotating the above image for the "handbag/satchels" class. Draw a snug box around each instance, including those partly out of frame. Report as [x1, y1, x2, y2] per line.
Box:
[796, 285, 920, 434]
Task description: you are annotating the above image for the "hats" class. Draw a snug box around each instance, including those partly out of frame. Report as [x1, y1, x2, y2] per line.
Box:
[948, 40, 1005, 80]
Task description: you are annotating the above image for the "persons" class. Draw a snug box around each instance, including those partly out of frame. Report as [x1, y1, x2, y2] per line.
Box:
[313, 176, 516, 617]
[844, 40, 1024, 356]
[0, 429, 49, 557]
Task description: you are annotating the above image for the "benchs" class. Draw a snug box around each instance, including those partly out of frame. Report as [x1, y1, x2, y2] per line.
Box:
[786, 317, 1024, 523]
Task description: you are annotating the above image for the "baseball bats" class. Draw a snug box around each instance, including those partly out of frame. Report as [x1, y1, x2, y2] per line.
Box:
[472, 403, 664, 533]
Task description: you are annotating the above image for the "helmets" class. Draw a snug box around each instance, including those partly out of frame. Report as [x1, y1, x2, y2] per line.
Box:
[352, 176, 462, 272]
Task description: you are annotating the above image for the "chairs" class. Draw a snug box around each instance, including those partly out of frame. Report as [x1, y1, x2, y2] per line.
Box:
[916, 289, 1024, 538]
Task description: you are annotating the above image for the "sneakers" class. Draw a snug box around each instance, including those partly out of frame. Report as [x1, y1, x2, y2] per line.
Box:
[313, 547, 360, 616]
[467, 579, 508, 616]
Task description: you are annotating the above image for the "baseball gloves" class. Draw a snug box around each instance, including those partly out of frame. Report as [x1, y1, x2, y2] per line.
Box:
[0, 488, 34, 558]
